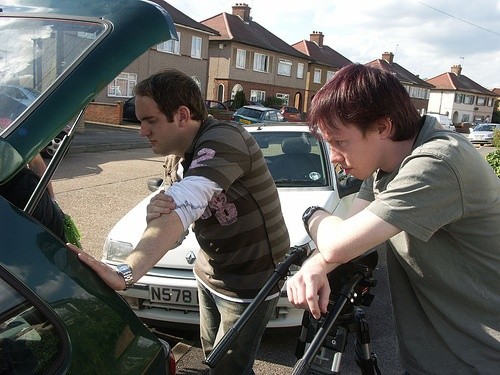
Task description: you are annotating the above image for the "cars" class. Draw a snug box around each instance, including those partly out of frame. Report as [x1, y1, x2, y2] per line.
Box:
[467, 123, 500, 146]
[122, 96, 302, 125]
[0, 0, 180, 375]
[0, 84, 72, 159]
[103, 125, 363, 329]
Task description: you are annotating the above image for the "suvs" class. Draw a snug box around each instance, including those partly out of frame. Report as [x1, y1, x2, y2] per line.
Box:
[427, 114, 456, 132]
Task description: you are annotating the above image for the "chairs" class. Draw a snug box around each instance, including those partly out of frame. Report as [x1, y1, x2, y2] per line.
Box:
[268, 136, 321, 180]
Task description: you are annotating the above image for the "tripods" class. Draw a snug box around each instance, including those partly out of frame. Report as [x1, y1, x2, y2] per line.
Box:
[205, 246, 383, 375]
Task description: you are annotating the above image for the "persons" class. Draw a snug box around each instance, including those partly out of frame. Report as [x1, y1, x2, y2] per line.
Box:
[0, 96, 67, 244]
[286, 62, 500, 375]
[67, 68, 290, 375]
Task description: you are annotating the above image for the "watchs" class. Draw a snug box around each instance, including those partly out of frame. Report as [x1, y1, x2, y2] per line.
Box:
[302, 206, 333, 241]
[115, 263, 134, 291]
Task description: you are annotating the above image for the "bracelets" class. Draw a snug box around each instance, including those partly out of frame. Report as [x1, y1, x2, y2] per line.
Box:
[51, 196, 56, 204]
